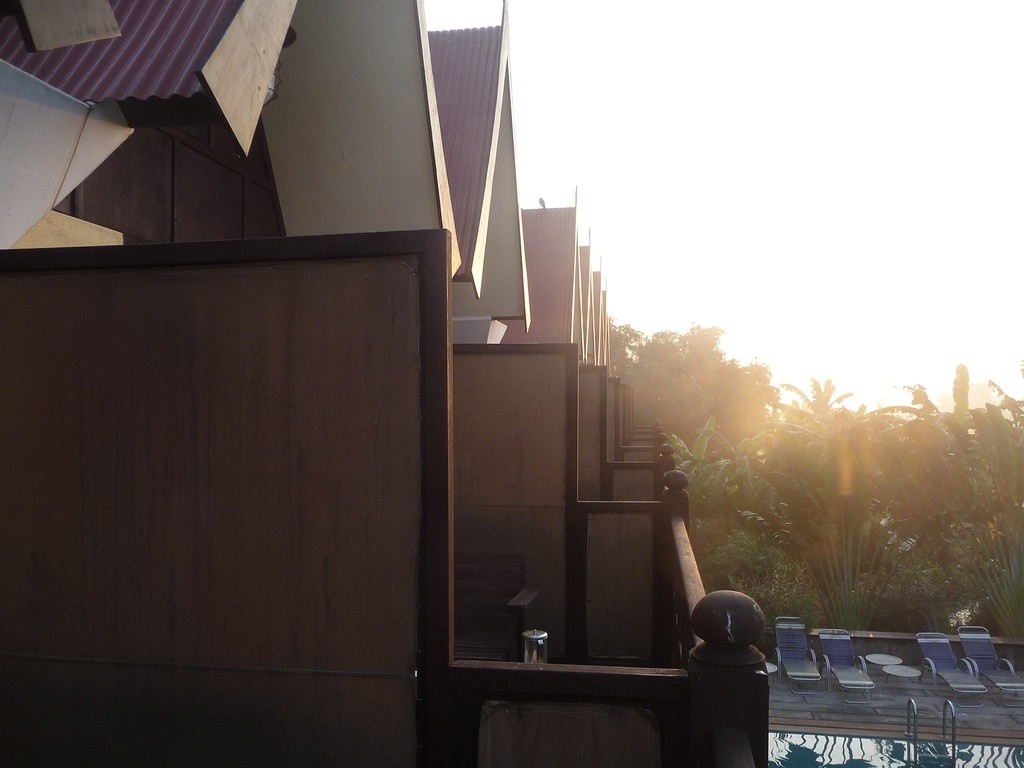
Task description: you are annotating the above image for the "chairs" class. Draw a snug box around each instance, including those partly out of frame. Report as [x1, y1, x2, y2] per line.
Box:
[772, 614, 823, 695]
[816, 627, 876, 704]
[957, 625, 1024, 708]
[914, 631, 989, 708]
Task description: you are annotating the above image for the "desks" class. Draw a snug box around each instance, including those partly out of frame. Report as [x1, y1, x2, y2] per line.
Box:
[882, 665, 922, 697]
[864, 653, 903, 684]
